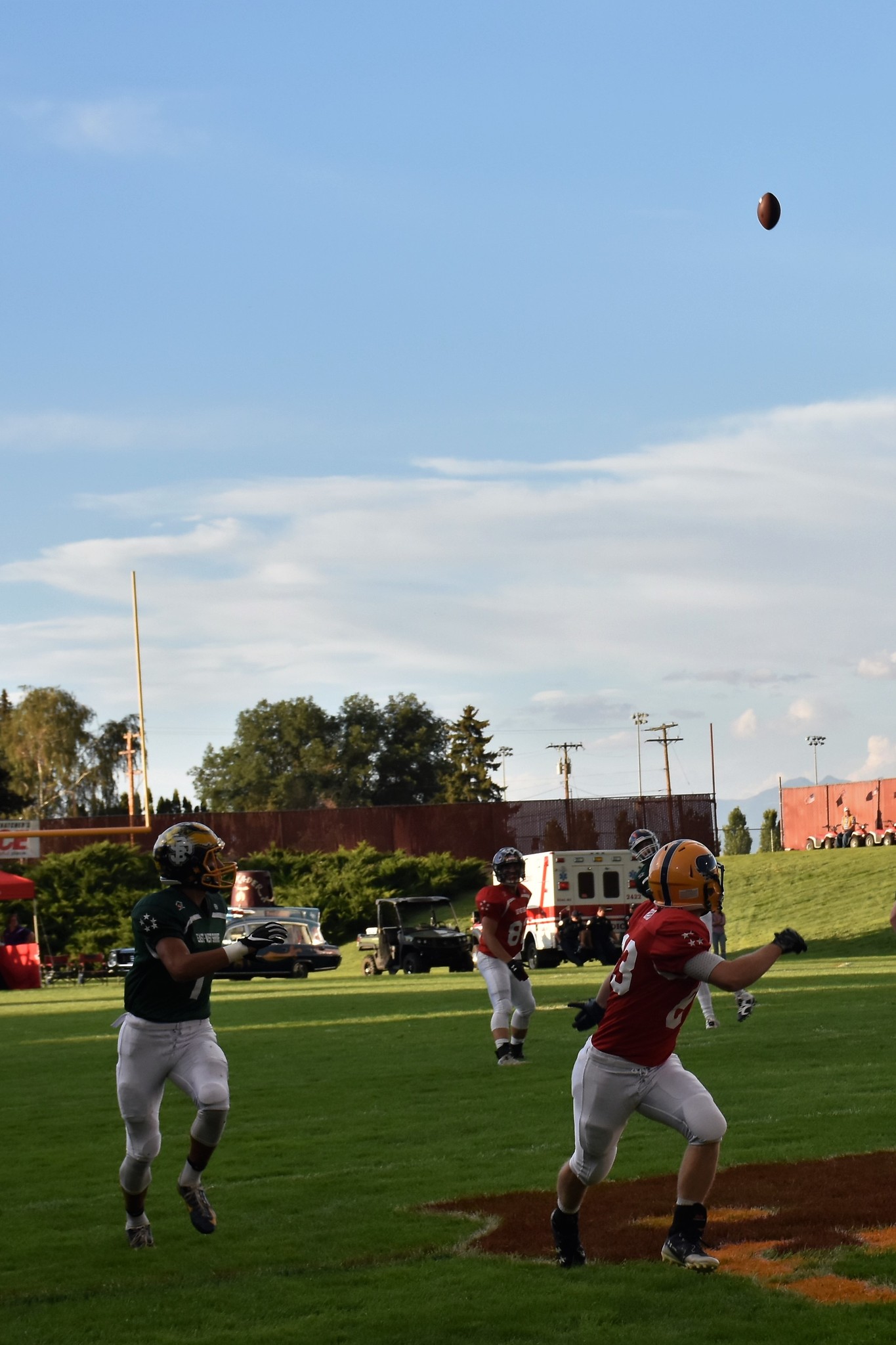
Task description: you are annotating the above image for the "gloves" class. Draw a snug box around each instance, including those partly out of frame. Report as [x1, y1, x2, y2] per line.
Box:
[507, 957, 530, 982]
[565, 997, 607, 1033]
[238, 921, 288, 960]
[772, 927, 807, 955]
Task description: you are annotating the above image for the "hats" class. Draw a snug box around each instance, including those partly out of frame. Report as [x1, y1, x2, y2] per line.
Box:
[571, 910, 583, 917]
[630, 902, 639, 909]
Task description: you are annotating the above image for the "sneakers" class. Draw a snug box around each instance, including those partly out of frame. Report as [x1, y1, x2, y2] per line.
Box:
[550, 1208, 587, 1270]
[706, 1020, 719, 1030]
[660, 1228, 720, 1274]
[177, 1175, 216, 1234]
[126, 1222, 153, 1250]
[734, 991, 757, 1022]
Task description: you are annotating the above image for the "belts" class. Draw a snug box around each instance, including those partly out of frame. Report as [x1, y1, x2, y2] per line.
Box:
[585, 1036, 644, 1068]
[110, 1012, 210, 1030]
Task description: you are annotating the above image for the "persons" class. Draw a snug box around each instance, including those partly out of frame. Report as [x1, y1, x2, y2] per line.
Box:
[629, 829, 758, 1030]
[111, 823, 287, 1247]
[475, 847, 536, 1066]
[550, 840, 808, 1270]
[712, 901, 727, 960]
[835, 807, 855, 848]
[555, 903, 639, 966]
[890, 899, 896, 931]
[0, 914, 35, 946]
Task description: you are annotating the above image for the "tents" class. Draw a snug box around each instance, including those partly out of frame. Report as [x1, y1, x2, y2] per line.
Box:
[0, 870, 39, 943]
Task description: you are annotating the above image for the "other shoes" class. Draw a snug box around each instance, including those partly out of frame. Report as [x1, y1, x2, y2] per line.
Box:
[498, 1054, 532, 1065]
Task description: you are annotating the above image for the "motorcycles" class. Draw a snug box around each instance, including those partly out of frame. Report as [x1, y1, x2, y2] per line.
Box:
[805, 819, 896, 850]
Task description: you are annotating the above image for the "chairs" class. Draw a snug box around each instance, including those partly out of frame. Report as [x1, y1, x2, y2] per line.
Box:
[78, 954, 109, 987]
[40, 955, 78, 988]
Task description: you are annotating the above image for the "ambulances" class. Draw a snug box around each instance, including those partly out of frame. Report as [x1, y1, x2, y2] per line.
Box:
[473, 850, 648, 970]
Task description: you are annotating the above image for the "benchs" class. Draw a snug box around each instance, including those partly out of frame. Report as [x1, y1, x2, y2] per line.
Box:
[383, 926, 419, 959]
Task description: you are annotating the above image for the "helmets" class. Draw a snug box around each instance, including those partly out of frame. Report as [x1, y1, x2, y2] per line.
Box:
[491, 847, 527, 887]
[628, 829, 660, 865]
[153, 821, 236, 897]
[648, 837, 724, 916]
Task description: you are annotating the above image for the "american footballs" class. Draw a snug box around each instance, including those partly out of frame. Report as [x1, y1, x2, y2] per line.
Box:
[757, 193, 781, 231]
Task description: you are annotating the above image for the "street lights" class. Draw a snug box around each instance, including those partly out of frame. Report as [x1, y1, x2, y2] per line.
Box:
[806, 736, 826, 785]
[496, 746, 514, 801]
[633, 713, 649, 797]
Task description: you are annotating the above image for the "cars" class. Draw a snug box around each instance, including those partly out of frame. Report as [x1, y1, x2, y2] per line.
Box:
[108, 915, 342, 981]
[361, 894, 478, 974]
